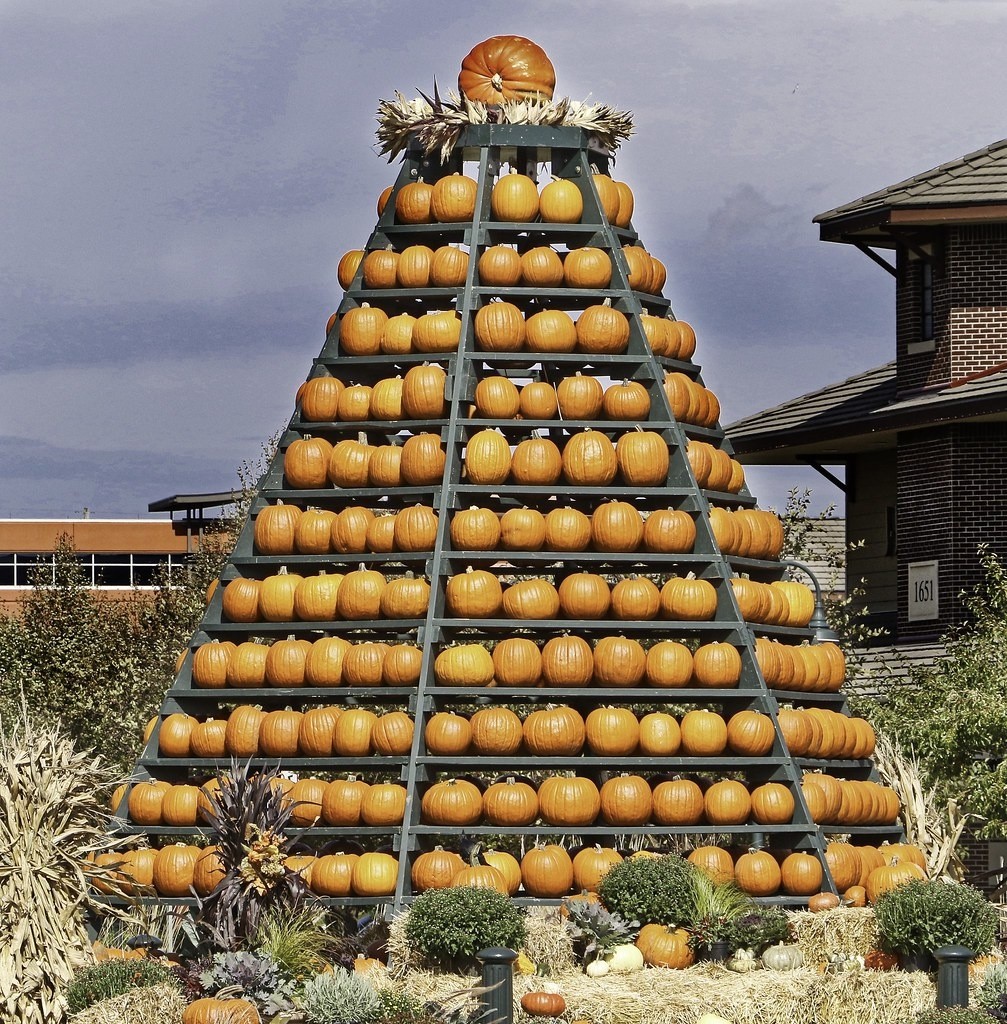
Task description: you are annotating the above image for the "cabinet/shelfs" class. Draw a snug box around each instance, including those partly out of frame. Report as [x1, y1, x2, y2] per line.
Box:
[90, 121, 910, 975]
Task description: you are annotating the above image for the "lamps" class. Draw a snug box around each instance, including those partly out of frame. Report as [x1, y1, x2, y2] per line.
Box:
[782, 561, 841, 644]
